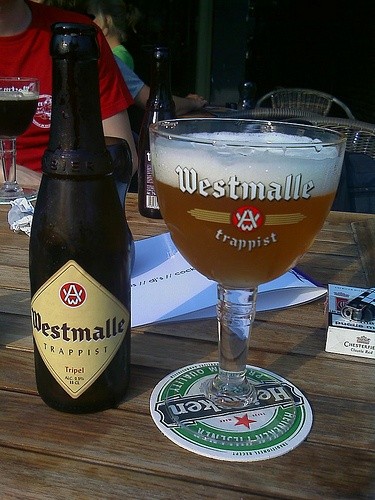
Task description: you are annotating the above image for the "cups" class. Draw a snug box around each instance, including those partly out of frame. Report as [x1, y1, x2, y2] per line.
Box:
[239, 79, 257, 111]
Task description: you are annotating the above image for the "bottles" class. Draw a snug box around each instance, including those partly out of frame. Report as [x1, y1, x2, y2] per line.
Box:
[137, 46, 177, 220]
[28, 21, 134, 416]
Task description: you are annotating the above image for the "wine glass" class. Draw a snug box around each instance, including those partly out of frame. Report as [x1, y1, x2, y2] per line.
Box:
[148, 116, 347, 446]
[0, 76, 40, 204]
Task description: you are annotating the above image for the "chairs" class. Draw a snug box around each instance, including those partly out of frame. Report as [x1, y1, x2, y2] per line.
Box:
[218, 88, 375, 215]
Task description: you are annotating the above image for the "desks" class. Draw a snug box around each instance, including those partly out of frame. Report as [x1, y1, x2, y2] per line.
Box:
[0, 183, 375, 500]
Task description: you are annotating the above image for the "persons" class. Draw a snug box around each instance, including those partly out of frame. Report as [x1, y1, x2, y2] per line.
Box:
[0, 0, 138, 191]
[113, 53, 208, 191]
[86, 0, 142, 71]
